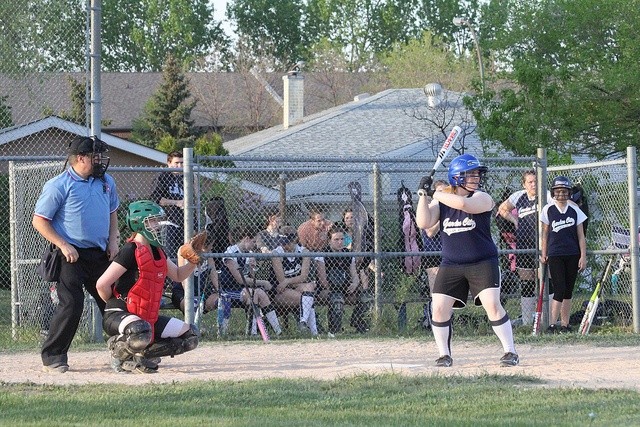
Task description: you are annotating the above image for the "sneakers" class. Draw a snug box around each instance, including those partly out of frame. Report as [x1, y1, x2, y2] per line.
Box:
[107, 335, 131, 375]
[132, 353, 159, 376]
[559, 325, 570, 334]
[45, 362, 70, 375]
[546, 324, 558, 334]
[435, 355, 453, 367]
[500, 352, 520, 368]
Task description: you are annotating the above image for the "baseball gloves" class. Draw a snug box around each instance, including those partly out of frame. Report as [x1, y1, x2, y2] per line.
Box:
[180, 230, 206, 265]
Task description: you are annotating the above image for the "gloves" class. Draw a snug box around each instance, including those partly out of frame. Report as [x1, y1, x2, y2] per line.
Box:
[417, 175, 436, 198]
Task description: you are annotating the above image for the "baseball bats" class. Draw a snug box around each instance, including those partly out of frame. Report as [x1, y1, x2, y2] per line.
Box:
[581, 258, 612, 336]
[418, 125, 462, 196]
[533, 257, 546, 335]
[578, 260, 610, 333]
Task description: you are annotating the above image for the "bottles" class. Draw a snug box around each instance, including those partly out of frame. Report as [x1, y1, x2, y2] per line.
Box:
[50, 286, 59, 305]
[610, 274, 618, 294]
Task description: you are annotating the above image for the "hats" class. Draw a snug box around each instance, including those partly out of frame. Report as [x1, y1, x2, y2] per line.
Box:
[275, 232, 298, 246]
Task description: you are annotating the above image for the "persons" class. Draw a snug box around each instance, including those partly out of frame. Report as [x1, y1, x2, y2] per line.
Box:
[151, 151, 195, 310]
[539, 176, 588, 334]
[171, 231, 231, 337]
[264, 224, 320, 340]
[493, 185, 519, 277]
[217, 224, 298, 340]
[298, 209, 334, 260]
[416, 180, 454, 330]
[38, 276, 61, 341]
[316, 226, 362, 340]
[499, 170, 554, 335]
[254, 207, 286, 274]
[30, 135, 121, 377]
[335, 208, 386, 332]
[194, 196, 233, 313]
[414, 155, 519, 368]
[96, 200, 207, 377]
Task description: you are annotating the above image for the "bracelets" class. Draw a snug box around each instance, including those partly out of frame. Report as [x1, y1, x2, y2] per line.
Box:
[427, 190, 436, 196]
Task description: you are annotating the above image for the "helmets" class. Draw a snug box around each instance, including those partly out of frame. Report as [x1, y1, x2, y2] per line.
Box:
[69, 135, 111, 177]
[126, 200, 179, 248]
[447, 154, 488, 192]
[550, 176, 572, 201]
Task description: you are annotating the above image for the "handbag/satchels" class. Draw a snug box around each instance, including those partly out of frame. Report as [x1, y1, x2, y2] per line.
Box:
[34, 242, 62, 282]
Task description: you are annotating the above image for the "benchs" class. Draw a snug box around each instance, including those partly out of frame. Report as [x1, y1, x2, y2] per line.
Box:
[158, 293, 525, 342]
